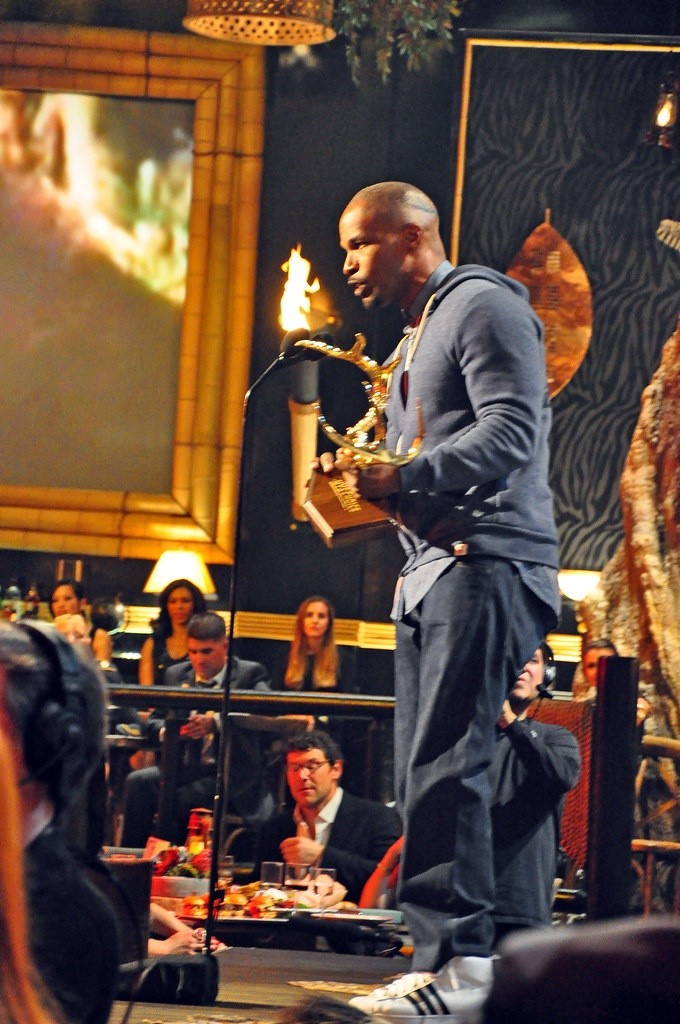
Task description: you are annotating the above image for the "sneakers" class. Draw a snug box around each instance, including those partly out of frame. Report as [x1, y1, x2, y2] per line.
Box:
[348, 954, 502, 1024]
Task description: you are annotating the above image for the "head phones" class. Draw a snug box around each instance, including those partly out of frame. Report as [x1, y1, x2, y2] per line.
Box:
[537, 641, 556, 688]
[14, 614, 87, 782]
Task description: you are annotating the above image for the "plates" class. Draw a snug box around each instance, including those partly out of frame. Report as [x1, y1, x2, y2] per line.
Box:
[214, 907, 393, 927]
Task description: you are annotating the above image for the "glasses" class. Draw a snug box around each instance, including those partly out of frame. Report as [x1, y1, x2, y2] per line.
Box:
[283, 759, 330, 779]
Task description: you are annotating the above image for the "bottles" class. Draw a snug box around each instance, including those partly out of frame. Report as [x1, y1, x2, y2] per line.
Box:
[217, 854, 234, 887]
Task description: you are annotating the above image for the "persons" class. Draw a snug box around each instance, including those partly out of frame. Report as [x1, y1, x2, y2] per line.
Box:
[0, 577, 401, 1024]
[489, 637, 580, 947]
[309, 182, 561, 1024]
[572, 637, 650, 769]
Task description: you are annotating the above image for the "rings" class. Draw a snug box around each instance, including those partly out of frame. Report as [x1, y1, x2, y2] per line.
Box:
[193, 730, 199, 736]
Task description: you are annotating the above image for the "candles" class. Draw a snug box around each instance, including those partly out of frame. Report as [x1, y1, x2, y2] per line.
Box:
[75, 559, 83, 581]
[58, 559, 64, 579]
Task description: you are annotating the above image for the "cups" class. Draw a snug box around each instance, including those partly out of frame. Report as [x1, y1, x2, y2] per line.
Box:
[260, 861, 283, 891]
[283, 863, 312, 892]
[313, 867, 337, 896]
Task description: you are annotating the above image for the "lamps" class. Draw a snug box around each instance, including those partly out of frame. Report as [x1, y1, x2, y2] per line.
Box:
[140, 549, 217, 596]
[644, 71, 679, 150]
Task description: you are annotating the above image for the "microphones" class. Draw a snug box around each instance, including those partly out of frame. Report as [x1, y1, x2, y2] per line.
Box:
[279, 329, 333, 362]
[537, 684, 553, 700]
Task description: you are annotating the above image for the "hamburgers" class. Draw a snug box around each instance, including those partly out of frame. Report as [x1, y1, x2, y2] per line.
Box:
[181, 894, 278, 919]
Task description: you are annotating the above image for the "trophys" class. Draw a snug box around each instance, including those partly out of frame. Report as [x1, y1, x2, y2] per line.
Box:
[292, 333, 425, 549]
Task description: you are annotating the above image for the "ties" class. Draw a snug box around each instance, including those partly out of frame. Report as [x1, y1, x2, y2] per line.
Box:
[188, 682, 217, 777]
